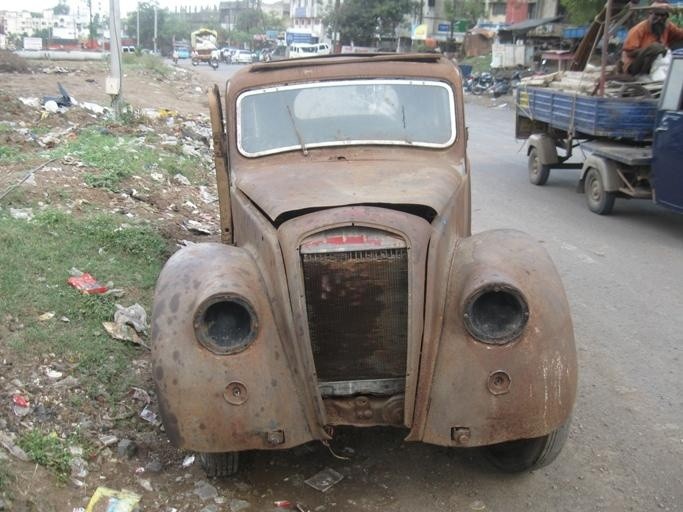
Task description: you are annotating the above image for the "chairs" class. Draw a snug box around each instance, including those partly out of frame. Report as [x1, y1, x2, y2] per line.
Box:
[243, 128, 300, 152]
[303, 113, 401, 141]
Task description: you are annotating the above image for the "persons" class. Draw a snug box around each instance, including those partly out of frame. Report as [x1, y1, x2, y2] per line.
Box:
[191, 48, 198, 59]
[173, 50, 180, 58]
[224, 48, 231, 57]
[622, 2, 683, 73]
[210, 49, 219, 60]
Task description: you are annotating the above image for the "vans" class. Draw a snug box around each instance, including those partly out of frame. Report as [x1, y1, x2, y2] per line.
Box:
[121, 46, 137, 55]
[290, 42, 330, 57]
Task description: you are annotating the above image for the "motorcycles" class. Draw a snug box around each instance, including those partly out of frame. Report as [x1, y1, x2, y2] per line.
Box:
[220, 49, 230, 65]
[514, 1, 682, 215]
[467, 69, 497, 96]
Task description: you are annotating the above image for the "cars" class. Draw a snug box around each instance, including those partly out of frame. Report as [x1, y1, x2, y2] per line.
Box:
[144, 54, 577, 482]
[232, 46, 285, 64]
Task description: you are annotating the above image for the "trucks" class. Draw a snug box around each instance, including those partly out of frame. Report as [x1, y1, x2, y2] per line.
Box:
[191, 27, 220, 67]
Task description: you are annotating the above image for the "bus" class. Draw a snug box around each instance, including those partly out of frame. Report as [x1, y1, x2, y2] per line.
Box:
[168, 43, 188, 58]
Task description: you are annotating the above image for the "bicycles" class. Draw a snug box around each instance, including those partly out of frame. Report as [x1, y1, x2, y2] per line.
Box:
[172, 53, 178, 65]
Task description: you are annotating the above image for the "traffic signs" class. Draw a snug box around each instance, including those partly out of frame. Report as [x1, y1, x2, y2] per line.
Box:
[437, 23, 449, 32]
